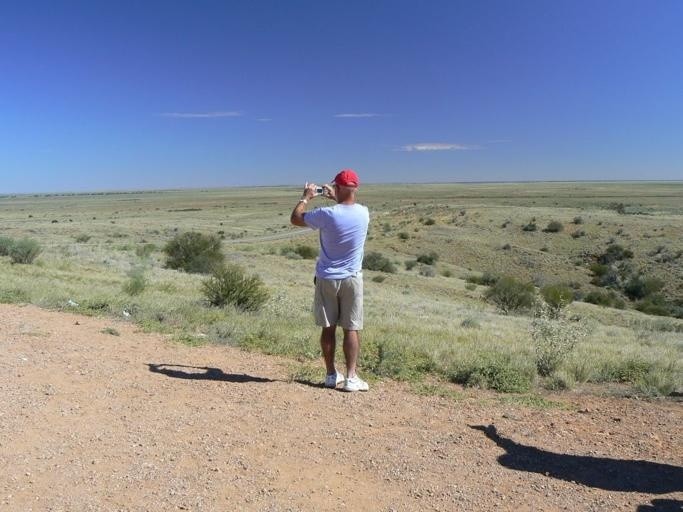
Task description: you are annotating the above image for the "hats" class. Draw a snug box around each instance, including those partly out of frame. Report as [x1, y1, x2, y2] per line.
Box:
[331, 169, 360, 188]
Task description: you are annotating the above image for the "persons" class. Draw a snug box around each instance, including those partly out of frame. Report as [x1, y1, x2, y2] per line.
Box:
[290, 168, 371, 392]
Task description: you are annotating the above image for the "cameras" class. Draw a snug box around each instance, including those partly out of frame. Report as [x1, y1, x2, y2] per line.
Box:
[315, 186, 323, 196]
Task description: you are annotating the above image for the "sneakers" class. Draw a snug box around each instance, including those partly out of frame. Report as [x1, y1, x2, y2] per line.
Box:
[323, 369, 344, 388]
[343, 375, 369, 391]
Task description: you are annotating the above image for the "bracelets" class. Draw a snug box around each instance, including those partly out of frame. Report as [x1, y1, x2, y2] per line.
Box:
[298, 198, 309, 205]
